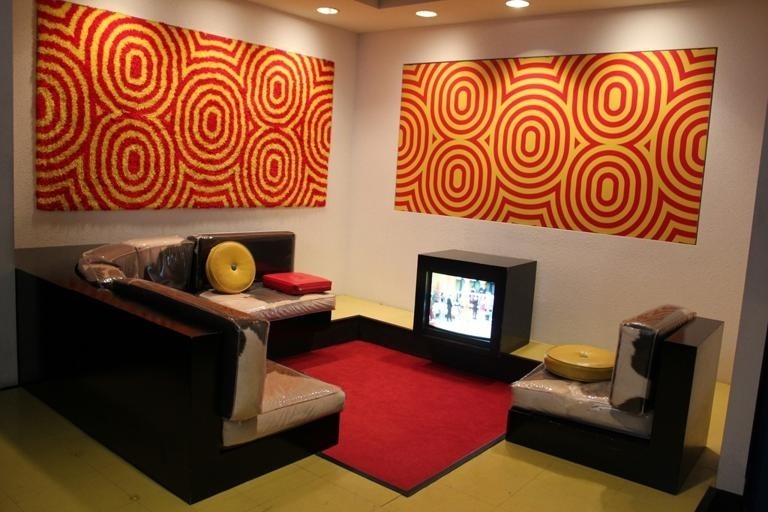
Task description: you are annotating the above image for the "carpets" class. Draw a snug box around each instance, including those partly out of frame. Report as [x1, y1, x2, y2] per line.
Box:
[270, 337, 510, 498]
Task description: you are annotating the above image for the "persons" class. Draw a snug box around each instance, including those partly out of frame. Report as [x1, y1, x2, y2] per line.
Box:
[429, 286, 491, 322]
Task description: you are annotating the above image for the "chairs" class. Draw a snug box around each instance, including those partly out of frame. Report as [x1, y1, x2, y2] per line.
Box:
[506, 304, 725, 495]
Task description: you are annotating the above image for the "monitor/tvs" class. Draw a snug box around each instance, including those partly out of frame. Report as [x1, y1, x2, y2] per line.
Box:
[411, 248, 536, 357]
